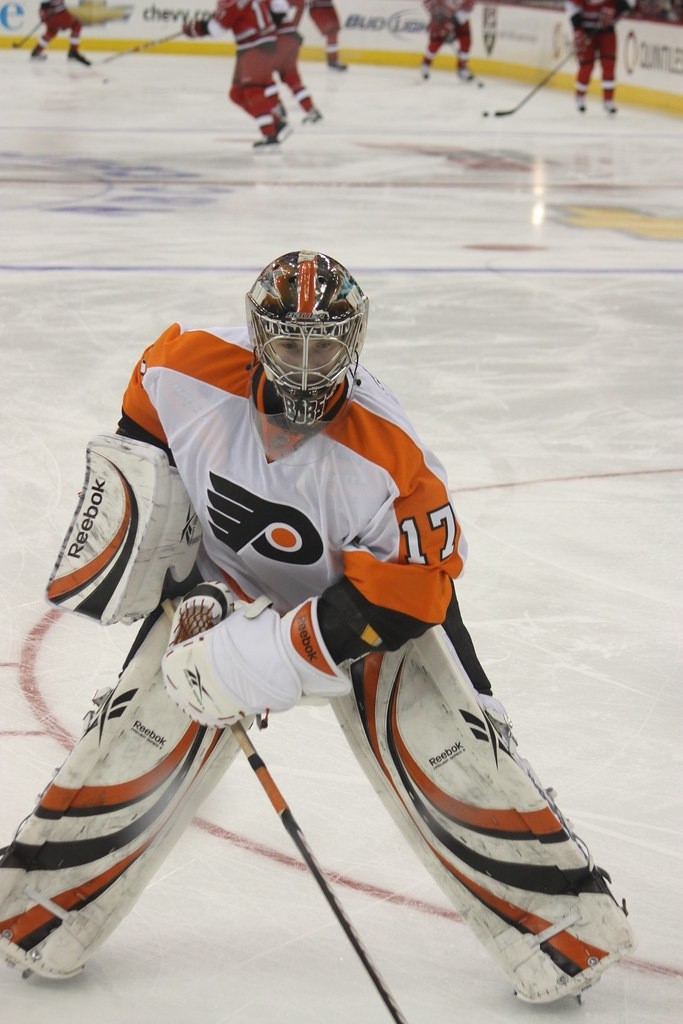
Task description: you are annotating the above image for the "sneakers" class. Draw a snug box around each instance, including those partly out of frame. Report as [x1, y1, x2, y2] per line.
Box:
[423, 65, 429, 75]
[253, 137, 279, 147]
[602, 100, 614, 110]
[303, 109, 321, 123]
[458, 69, 471, 78]
[69, 49, 84, 60]
[32, 50, 39, 57]
[277, 121, 286, 133]
[577, 96, 584, 108]
[329, 61, 347, 69]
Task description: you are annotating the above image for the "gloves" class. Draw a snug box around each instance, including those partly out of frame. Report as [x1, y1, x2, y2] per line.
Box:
[164, 582, 352, 729]
[183, 20, 210, 38]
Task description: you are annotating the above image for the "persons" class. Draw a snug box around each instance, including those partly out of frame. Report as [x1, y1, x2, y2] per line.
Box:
[421, 0, 477, 82]
[31, 0, 84, 60]
[182, 0, 347, 149]
[565, 0, 637, 115]
[0, 251, 639, 1006]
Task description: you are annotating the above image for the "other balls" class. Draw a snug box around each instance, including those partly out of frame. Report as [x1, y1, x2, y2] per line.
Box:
[483, 111, 489, 118]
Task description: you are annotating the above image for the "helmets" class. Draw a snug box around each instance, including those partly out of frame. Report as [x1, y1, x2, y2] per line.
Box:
[246, 250, 370, 424]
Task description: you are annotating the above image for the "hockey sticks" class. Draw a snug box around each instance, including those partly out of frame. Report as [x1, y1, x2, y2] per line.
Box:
[494, 49, 575, 118]
[12, 19, 43, 48]
[100, 31, 185, 64]
[163, 599, 411, 1024]
